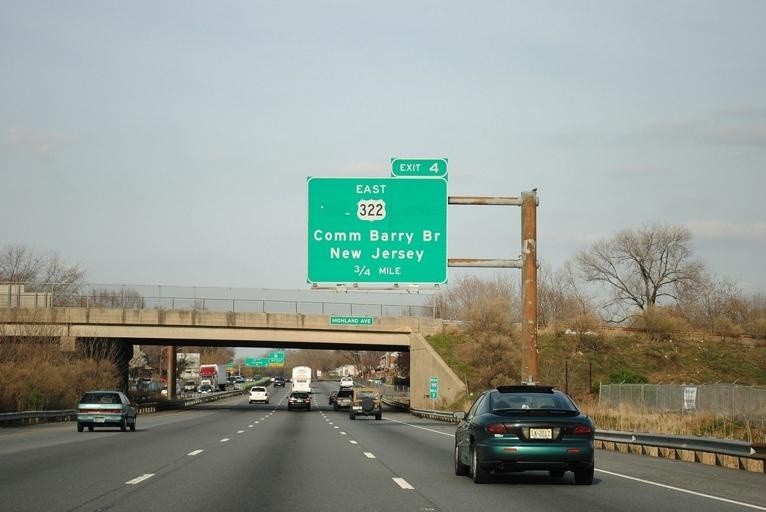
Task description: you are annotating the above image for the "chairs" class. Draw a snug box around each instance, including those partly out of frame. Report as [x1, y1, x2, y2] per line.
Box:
[494, 401, 511, 408]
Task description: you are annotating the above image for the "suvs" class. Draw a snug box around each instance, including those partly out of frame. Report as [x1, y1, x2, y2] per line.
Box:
[349, 388, 382, 420]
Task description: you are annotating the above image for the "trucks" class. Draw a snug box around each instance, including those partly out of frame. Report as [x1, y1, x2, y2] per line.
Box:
[199, 363, 226, 391]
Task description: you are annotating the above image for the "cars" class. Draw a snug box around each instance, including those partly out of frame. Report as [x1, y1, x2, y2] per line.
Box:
[339, 376, 353, 387]
[455, 381, 596, 485]
[248, 386, 269, 404]
[226, 375, 261, 386]
[329, 386, 353, 410]
[161, 380, 215, 397]
[287, 391, 311, 411]
[76, 390, 137, 433]
[273, 376, 289, 387]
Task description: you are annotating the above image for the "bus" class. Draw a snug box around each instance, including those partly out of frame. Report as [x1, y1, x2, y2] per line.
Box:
[292, 365, 312, 393]
